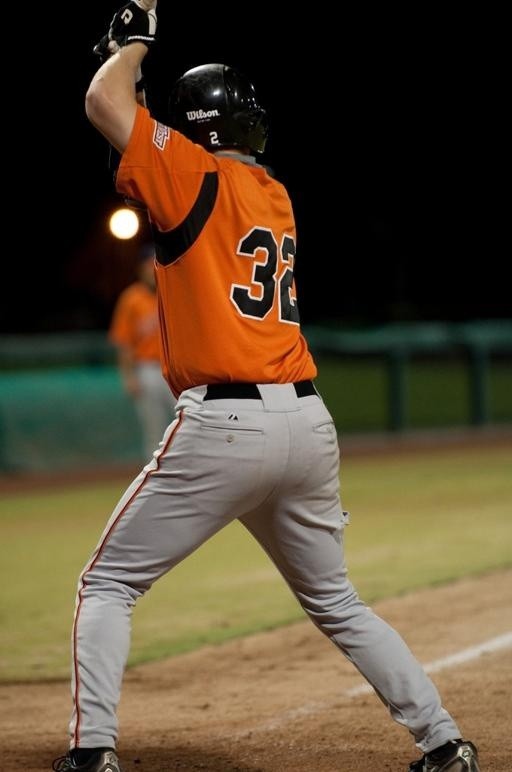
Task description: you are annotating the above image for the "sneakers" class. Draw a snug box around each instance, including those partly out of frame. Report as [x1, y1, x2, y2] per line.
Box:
[52, 748, 121, 771]
[409, 742, 480, 771]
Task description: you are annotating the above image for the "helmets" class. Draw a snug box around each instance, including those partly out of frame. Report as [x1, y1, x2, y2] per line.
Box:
[160, 63, 267, 156]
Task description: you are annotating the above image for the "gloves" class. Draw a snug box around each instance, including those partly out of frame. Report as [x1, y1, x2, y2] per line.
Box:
[93, 3, 161, 101]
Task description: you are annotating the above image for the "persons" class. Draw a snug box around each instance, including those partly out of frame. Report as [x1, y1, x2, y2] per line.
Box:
[49, 1, 482, 770]
[107, 251, 179, 468]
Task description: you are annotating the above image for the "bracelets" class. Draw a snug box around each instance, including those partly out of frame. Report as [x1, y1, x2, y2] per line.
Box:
[135, 76, 145, 95]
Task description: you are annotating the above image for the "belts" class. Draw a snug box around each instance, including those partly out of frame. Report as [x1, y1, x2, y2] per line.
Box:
[204, 377, 319, 400]
